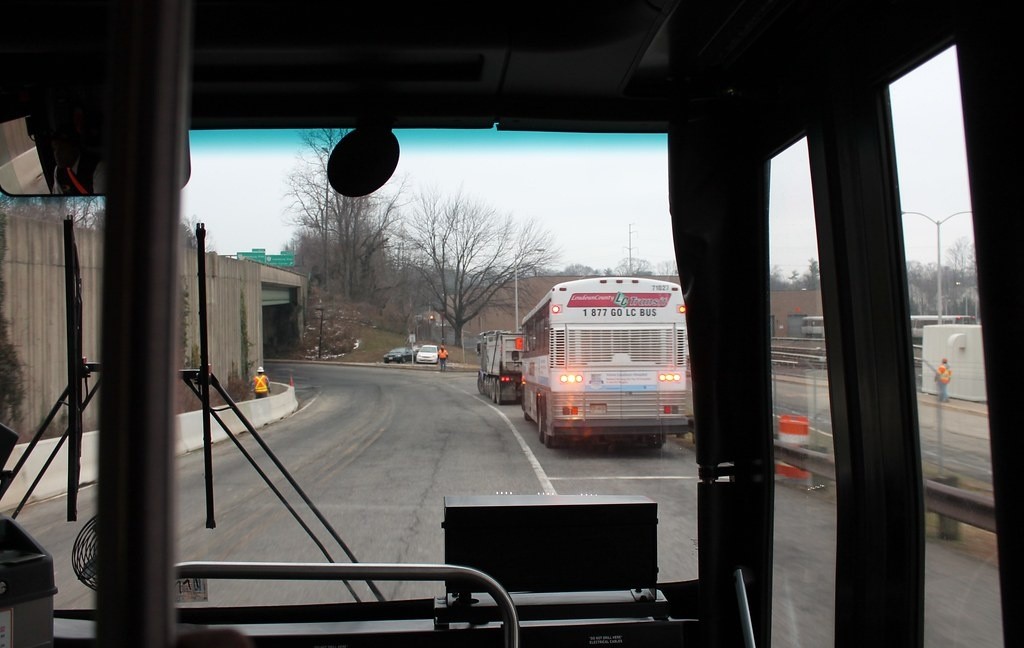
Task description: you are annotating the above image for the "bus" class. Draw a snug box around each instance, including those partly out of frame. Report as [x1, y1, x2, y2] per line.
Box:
[521, 276, 693, 456]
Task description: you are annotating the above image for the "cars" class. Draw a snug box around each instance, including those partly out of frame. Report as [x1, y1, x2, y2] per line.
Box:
[383, 346, 416, 364]
[416, 344, 440, 363]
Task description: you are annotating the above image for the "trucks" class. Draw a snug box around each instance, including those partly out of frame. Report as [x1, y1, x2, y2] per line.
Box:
[475, 330, 522, 404]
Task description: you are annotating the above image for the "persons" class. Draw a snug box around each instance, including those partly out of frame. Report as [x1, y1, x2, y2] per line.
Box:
[252, 366, 271, 399]
[934, 357, 952, 402]
[437, 345, 449, 371]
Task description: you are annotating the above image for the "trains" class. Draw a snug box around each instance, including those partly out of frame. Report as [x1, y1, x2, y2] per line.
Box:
[910, 315, 978, 342]
[800, 316, 825, 337]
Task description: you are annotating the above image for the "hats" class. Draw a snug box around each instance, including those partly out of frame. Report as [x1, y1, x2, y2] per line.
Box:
[942, 358, 948, 362]
[256, 366, 264, 373]
